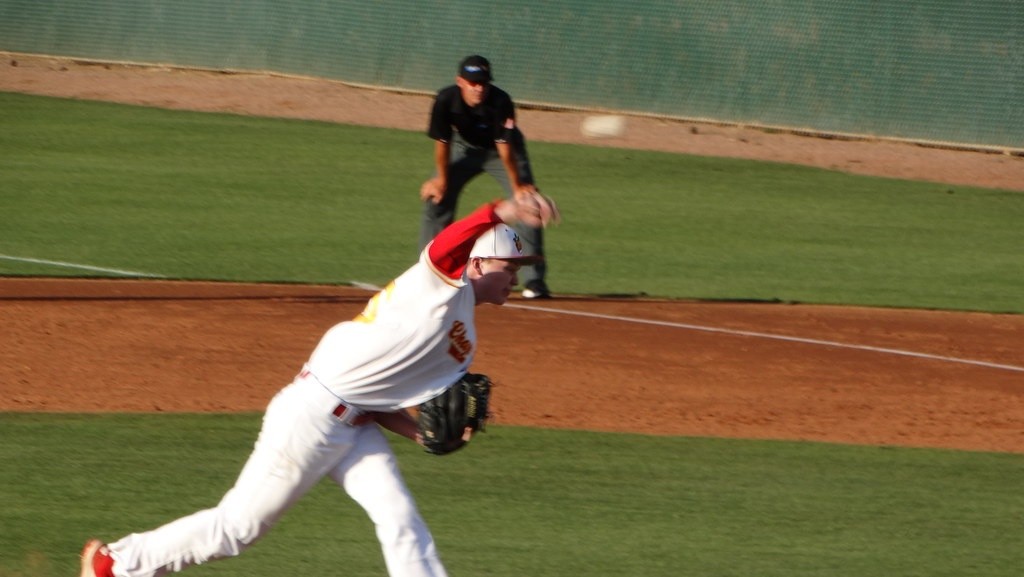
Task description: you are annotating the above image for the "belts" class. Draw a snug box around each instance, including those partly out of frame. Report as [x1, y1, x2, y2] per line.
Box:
[299, 371, 371, 427]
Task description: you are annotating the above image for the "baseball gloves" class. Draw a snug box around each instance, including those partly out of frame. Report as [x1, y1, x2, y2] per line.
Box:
[419, 374, 493, 456]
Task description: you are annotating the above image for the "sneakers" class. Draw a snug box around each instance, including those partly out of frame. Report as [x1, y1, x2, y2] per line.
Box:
[80, 538, 113, 577]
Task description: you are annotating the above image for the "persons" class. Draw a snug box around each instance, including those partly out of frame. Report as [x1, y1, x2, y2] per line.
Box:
[81, 188, 561, 577]
[418, 54, 553, 300]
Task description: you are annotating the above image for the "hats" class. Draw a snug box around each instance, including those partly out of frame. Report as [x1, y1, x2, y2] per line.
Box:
[459, 55, 495, 82]
[469, 223, 543, 265]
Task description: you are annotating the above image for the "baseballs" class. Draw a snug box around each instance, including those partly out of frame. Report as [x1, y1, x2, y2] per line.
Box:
[581, 115, 625, 138]
